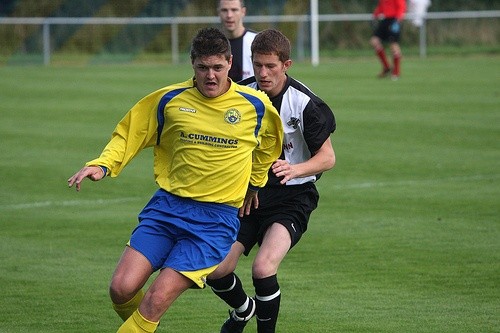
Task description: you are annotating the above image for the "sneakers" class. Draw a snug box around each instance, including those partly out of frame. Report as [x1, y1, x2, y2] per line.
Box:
[380, 69, 390, 78]
[392, 75, 399, 81]
[220, 296, 257, 333]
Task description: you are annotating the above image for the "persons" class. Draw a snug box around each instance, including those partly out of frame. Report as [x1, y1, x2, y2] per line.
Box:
[67, 25, 284, 333]
[369, 0, 407, 82]
[217, 0, 260, 90]
[206, 28, 337, 333]
